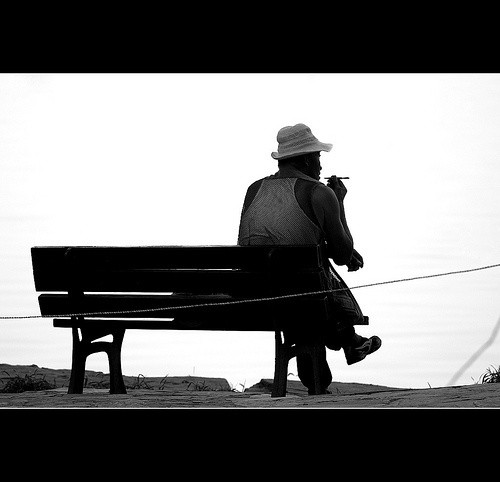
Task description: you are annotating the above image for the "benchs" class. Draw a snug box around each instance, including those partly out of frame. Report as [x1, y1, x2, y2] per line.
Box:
[30, 226, 373, 401]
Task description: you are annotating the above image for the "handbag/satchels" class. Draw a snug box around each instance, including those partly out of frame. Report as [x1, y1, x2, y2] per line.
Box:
[324, 280, 364, 323]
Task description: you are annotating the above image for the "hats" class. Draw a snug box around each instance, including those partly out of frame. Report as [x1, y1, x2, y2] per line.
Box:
[271, 124, 333, 161]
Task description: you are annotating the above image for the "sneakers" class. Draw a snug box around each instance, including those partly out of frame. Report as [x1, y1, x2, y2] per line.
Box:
[307, 388, 331, 396]
[345, 335, 382, 365]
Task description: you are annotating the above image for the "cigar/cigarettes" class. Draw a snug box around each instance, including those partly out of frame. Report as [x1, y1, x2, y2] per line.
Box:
[325, 177, 349, 180]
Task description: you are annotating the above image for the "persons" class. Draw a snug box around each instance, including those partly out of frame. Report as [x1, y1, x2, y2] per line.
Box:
[236, 123, 381, 396]
